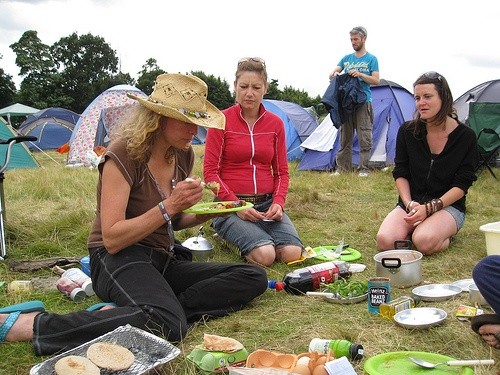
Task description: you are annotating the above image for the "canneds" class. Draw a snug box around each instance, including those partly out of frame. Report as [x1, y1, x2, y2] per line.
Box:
[7, 281, 32, 292]
[56, 268, 95, 301]
[368, 277, 391, 314]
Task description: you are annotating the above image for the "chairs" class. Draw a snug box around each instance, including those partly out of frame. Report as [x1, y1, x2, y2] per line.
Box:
[473, 128, 499, 182]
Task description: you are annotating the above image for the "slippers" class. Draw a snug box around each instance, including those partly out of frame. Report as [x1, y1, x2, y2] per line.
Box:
[85, 302, 119, 312]
[470, 314, 500, 349]
[0, 301, 46, 344]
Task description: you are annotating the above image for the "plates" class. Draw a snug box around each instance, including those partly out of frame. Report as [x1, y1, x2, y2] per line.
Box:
[394, 307, 447, 329]
[321, 288, 368, 303]
[181, 201, 254, 214]
[313, 246, 361, 261]
[451, 279, 475, 292]
[412, 284, 462, 301]
[364, 351, 475, 375]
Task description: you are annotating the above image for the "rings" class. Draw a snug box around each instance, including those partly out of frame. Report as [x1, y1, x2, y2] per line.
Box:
[412, 209, 415, 213]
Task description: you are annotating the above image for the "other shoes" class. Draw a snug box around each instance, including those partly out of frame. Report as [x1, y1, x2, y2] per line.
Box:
[330, 171, 340, 178]
[359, 173, 368, 177]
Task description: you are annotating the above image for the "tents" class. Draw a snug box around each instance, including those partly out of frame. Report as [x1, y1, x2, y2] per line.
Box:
[452, 80, 500, 169]
[190, 126, 207, 144]
[0, 115, 39, 168]
[67, 85, 149, 167]
[0, 102, 41, 126]
[262, 99, 319, 153]
[286, 80, 418, 171]
[20, 107, 81, 125]
[16, 118, 75, 149]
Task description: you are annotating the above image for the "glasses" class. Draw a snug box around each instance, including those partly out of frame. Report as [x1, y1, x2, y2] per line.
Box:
[237, 57, 266, 72]
[415, 71, 442, 81]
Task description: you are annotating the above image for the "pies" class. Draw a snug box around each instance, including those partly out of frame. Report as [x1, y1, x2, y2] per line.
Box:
[87, 342, 135, 370]
[203, 334, 243, 353]
[55, 354, 101, 375]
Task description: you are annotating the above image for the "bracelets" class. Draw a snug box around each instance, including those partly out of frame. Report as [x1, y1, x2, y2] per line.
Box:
[159, 201, 169, 222]
[406, 200, 414, 213]
[424, 199, 443, 217]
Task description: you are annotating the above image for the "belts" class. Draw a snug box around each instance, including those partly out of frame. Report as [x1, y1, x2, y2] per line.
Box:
[238, 194, 273, 206]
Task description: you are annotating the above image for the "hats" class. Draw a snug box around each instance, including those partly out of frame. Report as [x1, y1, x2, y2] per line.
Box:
[127, 73, 226, 131]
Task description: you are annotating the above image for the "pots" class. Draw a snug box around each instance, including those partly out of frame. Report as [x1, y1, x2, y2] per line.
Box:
[181, 225, 215, 257]
[374, 240, 423, 288]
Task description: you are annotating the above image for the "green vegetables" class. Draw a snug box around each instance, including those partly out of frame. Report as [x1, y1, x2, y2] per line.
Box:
[319, 276, 369, 298]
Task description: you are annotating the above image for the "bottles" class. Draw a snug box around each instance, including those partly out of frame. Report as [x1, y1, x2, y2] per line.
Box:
[7, 280, 33, 293]
[56, 268, 96, 303]
[379, 295, 421, 318]
[309, 337, 364, 362]
[268, 261, 352, 296]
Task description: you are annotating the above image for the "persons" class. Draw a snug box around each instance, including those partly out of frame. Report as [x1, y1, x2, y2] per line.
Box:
[204, 57, 304, 268]
[0, 73, 268, 355]
[472, 255, 500, 349]
[329, 27, 379, 178]
[376, 71, 479, 255]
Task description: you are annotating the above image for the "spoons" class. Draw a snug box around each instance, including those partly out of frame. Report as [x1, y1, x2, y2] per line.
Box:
[408, 356, 494, 368]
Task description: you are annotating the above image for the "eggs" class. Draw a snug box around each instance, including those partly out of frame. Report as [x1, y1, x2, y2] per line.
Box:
[293, 356, 329, 375]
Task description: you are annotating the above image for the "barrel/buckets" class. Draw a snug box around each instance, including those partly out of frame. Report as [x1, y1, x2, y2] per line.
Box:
[368, 277, 390, 312]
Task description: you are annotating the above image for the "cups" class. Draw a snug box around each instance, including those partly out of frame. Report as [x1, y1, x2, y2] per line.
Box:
[469, 285, 481, 304]
